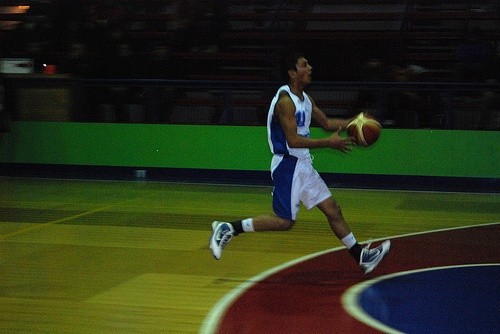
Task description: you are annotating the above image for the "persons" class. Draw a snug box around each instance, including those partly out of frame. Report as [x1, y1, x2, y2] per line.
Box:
[208, 54, 393, 275]
[0, 0, 500, 130]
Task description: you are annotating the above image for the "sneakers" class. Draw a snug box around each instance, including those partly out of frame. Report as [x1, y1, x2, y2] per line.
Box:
[359, 238, 390, 278]
[210, 220, 235, 260]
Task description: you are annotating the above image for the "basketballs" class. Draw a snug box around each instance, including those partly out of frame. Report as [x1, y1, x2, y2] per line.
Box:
[346, 111, 382, 146]
